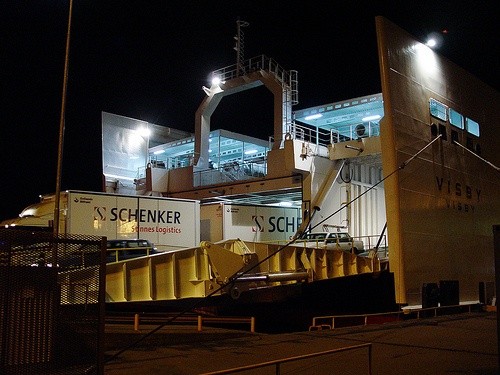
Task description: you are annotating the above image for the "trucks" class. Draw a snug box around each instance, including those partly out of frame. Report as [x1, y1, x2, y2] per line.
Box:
[1, 189, 200, 255]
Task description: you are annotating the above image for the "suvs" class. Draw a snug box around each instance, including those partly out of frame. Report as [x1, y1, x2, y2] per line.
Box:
[30, 240, 159, 269]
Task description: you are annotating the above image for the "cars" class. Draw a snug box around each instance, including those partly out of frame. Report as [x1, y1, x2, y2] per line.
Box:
[288, 232, 365, 256]
